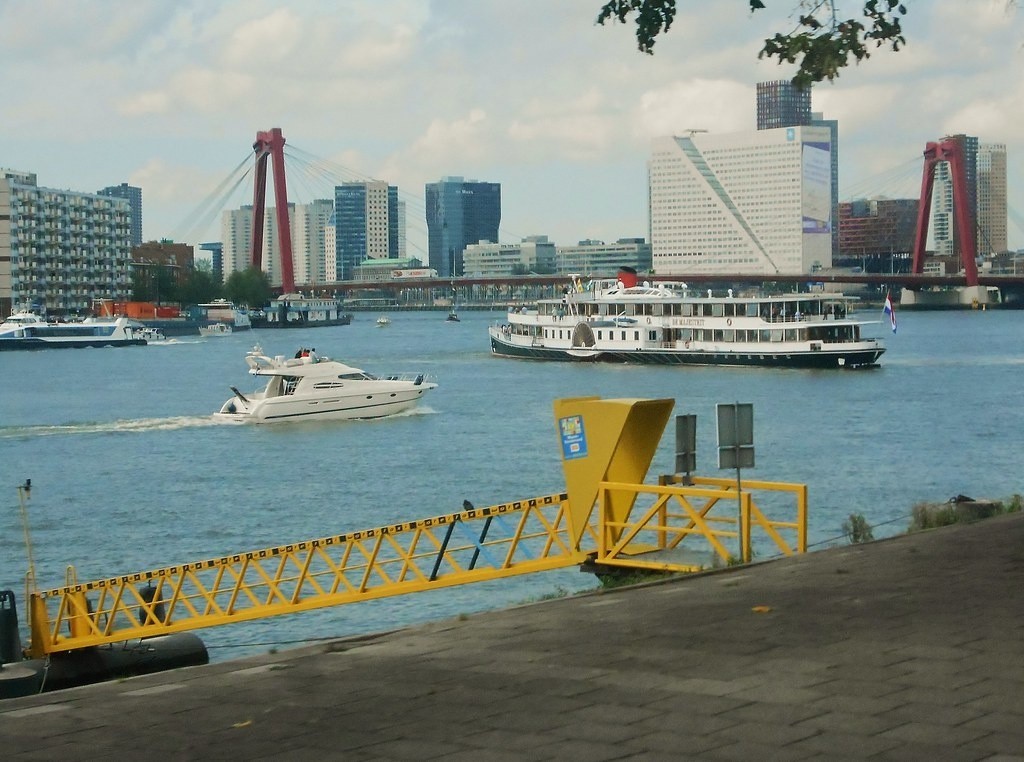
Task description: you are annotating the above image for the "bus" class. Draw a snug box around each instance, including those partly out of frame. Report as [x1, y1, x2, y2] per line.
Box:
[390, 268, 439, 278]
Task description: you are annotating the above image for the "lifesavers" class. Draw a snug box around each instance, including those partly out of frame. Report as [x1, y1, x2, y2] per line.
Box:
[685, 342, 691, 349]
[726, 318, 732, 325]
[645, 316, 652, 324]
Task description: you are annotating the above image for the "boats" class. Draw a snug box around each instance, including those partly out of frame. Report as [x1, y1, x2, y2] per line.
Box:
[376, 316, 392, 326]
[489, 281, 889, 371]
[198, 321, 233, 338]
[213, 342, 438, 427]
[239, 292, 356, 329]
[0, 298, 148, 349]
[446, 313, 460, 323]
[133, 327, 167, 341]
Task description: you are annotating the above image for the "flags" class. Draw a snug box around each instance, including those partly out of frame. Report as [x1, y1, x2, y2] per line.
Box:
[883, 295, 897, 333]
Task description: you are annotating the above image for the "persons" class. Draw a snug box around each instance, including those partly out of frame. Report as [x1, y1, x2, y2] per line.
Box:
[552, 306, 564, 322]
[762, 305, 806, 322]
[502, 323, 511, 336]
[508, 306, 528, 315]
[823, 309, 828, 320]
[295, 347, 317, 359]
